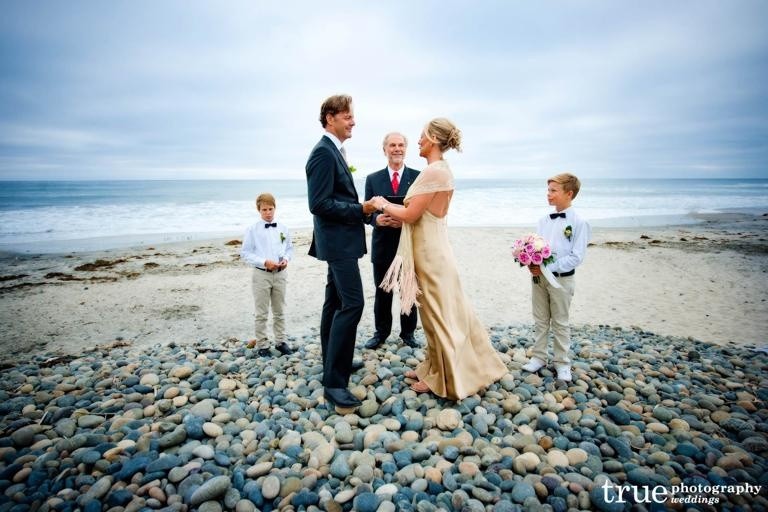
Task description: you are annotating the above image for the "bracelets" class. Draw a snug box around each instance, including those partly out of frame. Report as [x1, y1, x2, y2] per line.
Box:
[382, 202, 390, 212]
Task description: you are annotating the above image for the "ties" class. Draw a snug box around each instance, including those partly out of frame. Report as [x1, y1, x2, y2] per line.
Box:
[340, 146, 348, 166]
[391, 171, 400, 195]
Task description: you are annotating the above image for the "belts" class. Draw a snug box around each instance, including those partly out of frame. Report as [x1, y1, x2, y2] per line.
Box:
[255, 266, 282, 273]
[552, 269, 575, 278]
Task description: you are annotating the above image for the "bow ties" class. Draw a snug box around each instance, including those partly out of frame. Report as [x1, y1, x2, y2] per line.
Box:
[549, 212, 566, 219]
[265, 223, 277, 228]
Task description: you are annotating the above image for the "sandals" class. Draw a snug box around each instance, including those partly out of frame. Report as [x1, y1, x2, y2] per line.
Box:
[409, 380, 431, 393]
[403, 370, 418, 379]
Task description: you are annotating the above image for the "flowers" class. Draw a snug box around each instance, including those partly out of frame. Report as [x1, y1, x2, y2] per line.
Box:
[347, 161, 357, 172]
[511, 233, 555, 284]
[280, 231, 287, 244]
[564, 225, 572, 241]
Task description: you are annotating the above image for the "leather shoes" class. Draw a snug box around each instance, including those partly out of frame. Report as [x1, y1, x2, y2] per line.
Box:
[554, 364, 572, 383]
[350, 361, 365, 374]
[520, 355, 548, 373]
[258, 348, 273, 358]
[364, 336, 386, 349]
[275, 341, 294, 355]
[323, 385, 364, 409]
[402, 337, 420, 348]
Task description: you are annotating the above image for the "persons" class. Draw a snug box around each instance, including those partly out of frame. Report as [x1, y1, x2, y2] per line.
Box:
[238, 192, 294, 359]
[366, 115, 509, 396]
[363, 130, 425, 348]
[303, 94, 381, 408]
[520, 172, 592, 382]
